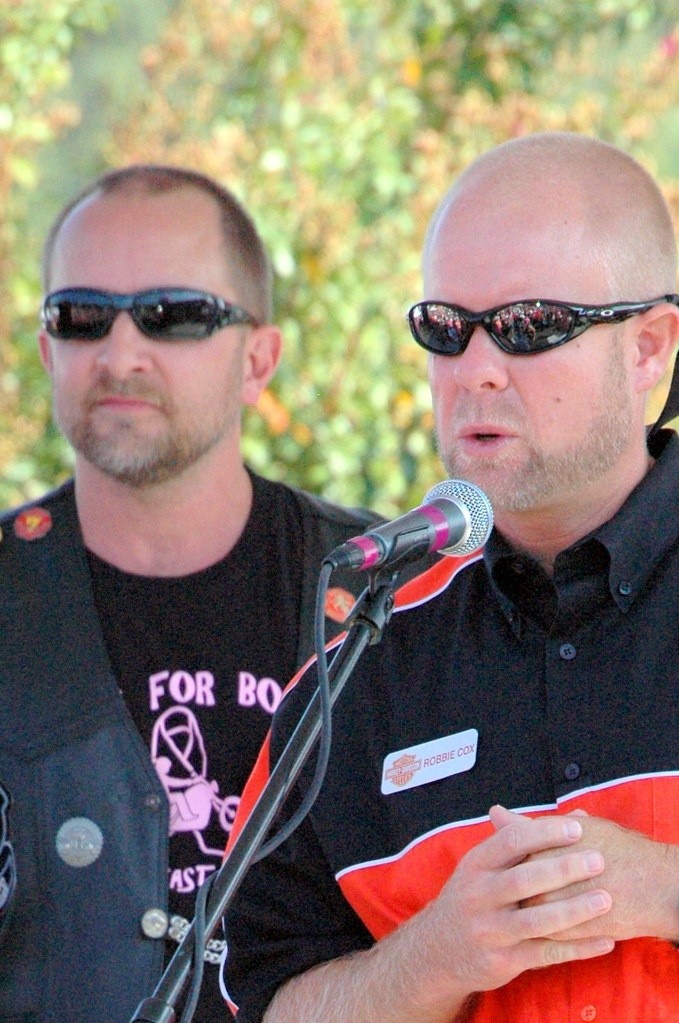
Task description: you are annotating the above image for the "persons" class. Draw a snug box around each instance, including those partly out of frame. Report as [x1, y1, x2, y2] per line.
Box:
[0, 165, 393, 1023]
[192, 134, 679, 1023]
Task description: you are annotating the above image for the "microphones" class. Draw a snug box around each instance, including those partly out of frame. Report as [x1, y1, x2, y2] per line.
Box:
[324, 480, 495, 571]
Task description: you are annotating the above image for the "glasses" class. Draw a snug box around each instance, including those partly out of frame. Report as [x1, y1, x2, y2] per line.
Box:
[41, 286, 258, 342]
[408, 295, 678, 356]
